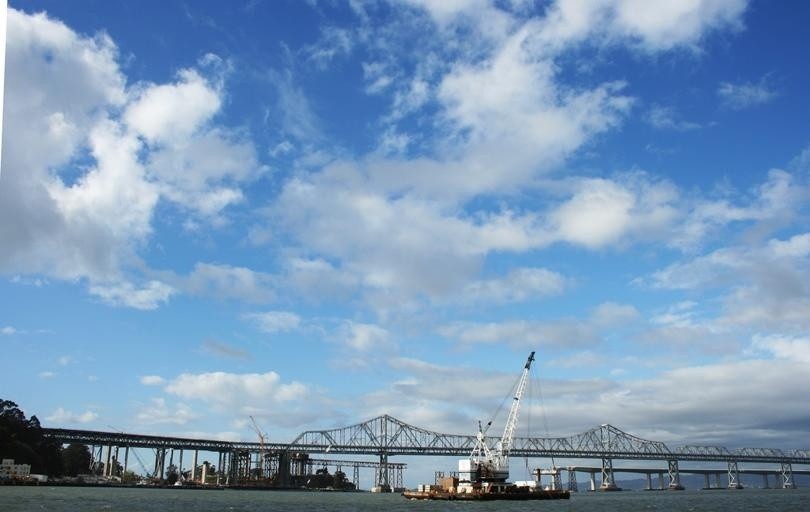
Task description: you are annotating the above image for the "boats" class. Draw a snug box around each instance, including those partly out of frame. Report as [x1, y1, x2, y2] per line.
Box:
[171, 477, 225, 489]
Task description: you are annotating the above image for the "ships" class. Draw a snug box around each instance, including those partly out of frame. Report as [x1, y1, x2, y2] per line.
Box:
[402, 351, 571, 500]
